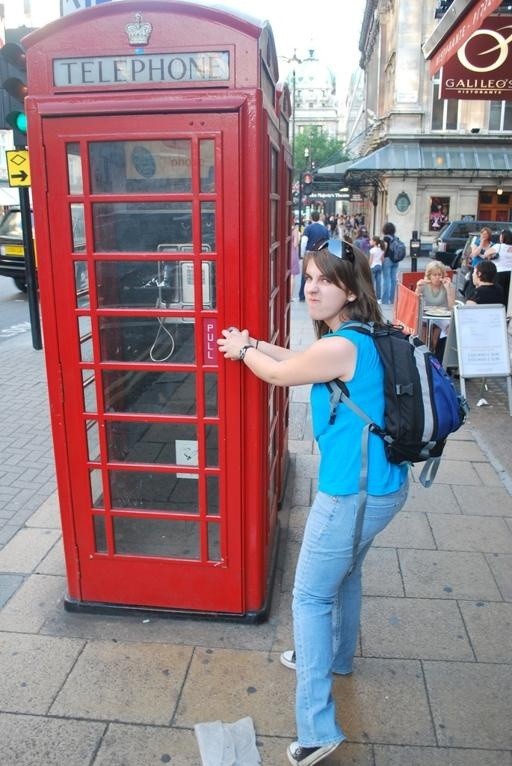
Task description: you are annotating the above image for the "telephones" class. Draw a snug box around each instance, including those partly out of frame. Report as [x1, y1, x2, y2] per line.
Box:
[157, 244, 211, 323]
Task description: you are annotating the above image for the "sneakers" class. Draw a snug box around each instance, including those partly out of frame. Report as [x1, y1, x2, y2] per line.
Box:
[285, 741, 342, 765]
[280, 651, 296, 667]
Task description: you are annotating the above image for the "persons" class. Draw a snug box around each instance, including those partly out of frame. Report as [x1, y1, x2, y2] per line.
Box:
[216, 239, 409, 766]
[290, 209, 512, 385]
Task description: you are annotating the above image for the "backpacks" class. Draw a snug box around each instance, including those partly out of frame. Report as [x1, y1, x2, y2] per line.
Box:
[384, 235, 405, 264]
[319, 322, 466, 466]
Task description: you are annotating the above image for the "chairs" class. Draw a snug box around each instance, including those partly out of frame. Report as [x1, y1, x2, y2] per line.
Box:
[394, 269, 511, 371]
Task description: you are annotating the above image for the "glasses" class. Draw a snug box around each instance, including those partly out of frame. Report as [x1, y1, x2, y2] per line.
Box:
[310, 240, 353, 261]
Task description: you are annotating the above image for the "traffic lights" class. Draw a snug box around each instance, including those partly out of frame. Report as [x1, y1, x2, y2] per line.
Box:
[303, 174, 312, 195]
[0, 42, 27, 137]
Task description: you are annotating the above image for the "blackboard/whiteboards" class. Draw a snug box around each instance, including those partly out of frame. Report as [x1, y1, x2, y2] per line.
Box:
[455, 304, 510, 378]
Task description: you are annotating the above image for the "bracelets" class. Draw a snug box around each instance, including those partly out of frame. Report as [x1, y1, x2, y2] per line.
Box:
[255, 340, 259, 350]
[239, 344, 253, 360]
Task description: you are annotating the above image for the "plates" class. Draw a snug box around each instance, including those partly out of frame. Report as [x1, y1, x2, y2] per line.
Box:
[424, 304, 448, 311]
[424, 311, 453, 317]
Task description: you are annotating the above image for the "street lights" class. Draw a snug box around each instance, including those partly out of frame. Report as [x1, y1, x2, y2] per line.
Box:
[277, 47, 319, 166]
[304, 131, 314, 172]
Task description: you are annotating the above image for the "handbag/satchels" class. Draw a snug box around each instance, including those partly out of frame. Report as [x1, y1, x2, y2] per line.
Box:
[490, 253, 499, 260]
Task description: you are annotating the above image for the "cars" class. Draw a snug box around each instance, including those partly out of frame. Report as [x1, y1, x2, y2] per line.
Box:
[0, 204, 88, 294]
[429, 220, 512, 270]
